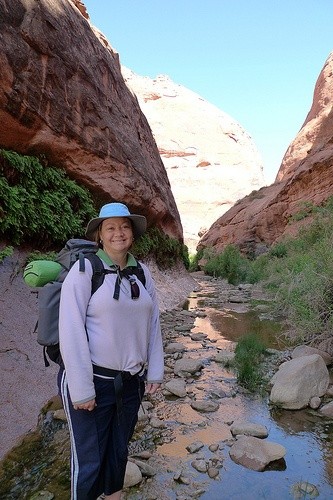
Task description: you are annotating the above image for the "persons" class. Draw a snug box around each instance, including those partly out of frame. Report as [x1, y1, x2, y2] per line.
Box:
[57, 203, 164, 500]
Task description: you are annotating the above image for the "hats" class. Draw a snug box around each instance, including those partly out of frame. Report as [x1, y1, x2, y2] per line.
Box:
[85, 203, 147, 244]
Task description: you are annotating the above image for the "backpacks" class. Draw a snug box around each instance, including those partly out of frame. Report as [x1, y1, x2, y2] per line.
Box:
[23, 239, 147, 383]
